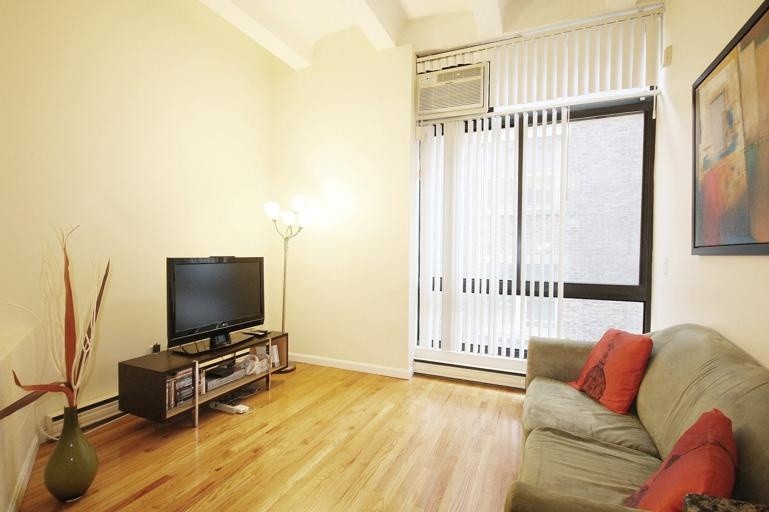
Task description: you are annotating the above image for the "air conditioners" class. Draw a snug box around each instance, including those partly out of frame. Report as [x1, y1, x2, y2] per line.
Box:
[415, 61, 489, 121]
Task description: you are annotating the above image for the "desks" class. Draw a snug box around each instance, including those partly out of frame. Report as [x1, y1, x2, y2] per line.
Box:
[118, 329, 288, 427]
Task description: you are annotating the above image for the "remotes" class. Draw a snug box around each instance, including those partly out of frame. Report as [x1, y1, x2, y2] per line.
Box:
[244, 331, 265, 337]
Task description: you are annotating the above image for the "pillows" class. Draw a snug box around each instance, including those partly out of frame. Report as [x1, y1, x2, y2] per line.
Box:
[622, 408, 740, 512]
[568, 328, 653, 414]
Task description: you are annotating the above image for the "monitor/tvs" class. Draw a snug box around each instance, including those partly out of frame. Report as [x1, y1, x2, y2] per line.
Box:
[167, 256, 264, 356]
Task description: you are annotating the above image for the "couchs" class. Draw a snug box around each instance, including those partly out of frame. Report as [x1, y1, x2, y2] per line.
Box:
[504, 323, 769, 512]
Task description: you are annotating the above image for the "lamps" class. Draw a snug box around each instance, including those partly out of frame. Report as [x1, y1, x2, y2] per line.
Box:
[267, 198, 311, 373]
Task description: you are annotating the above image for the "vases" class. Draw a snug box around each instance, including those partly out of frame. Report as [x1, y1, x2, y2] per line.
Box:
[45, 406, 98, 503]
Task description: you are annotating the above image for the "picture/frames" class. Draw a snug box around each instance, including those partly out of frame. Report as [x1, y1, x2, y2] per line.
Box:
[691, 0, 769, 255]
